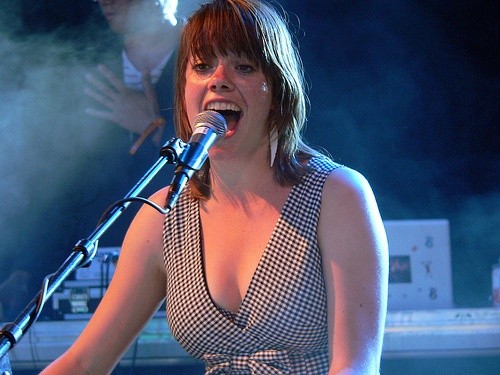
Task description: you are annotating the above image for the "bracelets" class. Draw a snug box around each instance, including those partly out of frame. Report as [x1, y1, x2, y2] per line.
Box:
[129, 116, 166, 155]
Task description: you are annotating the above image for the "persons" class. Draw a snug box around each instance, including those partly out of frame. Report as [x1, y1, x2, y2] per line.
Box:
[38, 0, 390, 375]
[1, 0, 193, 324]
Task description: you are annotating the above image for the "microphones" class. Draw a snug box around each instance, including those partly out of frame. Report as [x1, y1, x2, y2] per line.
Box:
[164, 109, 227, 210]
